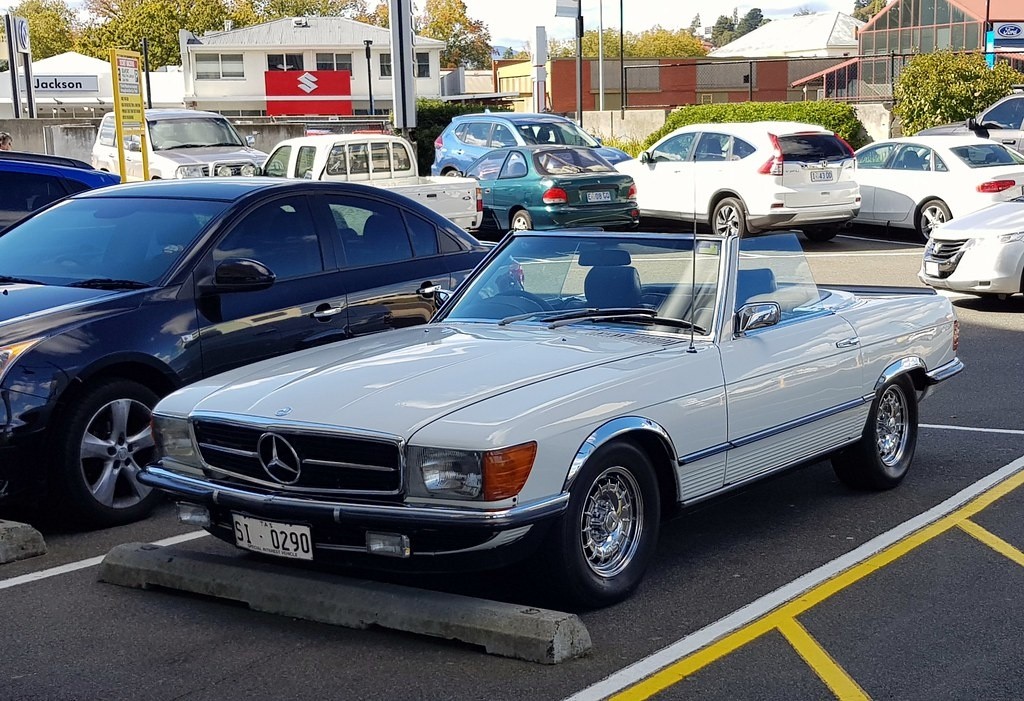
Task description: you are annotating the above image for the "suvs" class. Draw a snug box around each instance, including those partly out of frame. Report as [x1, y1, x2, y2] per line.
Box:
[90, 107, 284, 181]
[613, 120, 861, 243]
[915, 95, 1024, 158]
[430, 109, 634, 178]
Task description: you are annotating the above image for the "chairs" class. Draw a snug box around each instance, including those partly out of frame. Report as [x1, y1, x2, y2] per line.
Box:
[734, 269, 777, 313]
[941, 149, 973, 168]
[562, 266, 656, 310]
[896, 151, 923, 168]
[507, 162, 525, 175]
[698, 139, 722, 160]
[524, 127, 555, 143]
[145, 213, 202, 274]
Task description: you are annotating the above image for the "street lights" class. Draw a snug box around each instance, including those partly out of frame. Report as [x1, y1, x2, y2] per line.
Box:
[364, 39, 374, 115]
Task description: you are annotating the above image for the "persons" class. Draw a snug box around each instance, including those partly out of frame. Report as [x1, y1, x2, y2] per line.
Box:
[164, 243, 256, 261]
[0, 131, 14, 150]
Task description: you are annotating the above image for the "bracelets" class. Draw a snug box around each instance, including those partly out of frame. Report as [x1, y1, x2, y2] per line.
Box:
[178, 245, 184, 252]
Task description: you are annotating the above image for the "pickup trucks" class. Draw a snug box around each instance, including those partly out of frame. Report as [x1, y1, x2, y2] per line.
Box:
[253, 134, 483, 231]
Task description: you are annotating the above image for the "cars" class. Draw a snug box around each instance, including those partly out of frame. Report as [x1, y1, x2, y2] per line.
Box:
[917, 195, 1024, 300]
[133, 228, 965, 610]
[835, 135, 1024, 244]
[0, 177, 526, 532]
[0, 148, 122, 233]
[453, 145, 641, 232]
